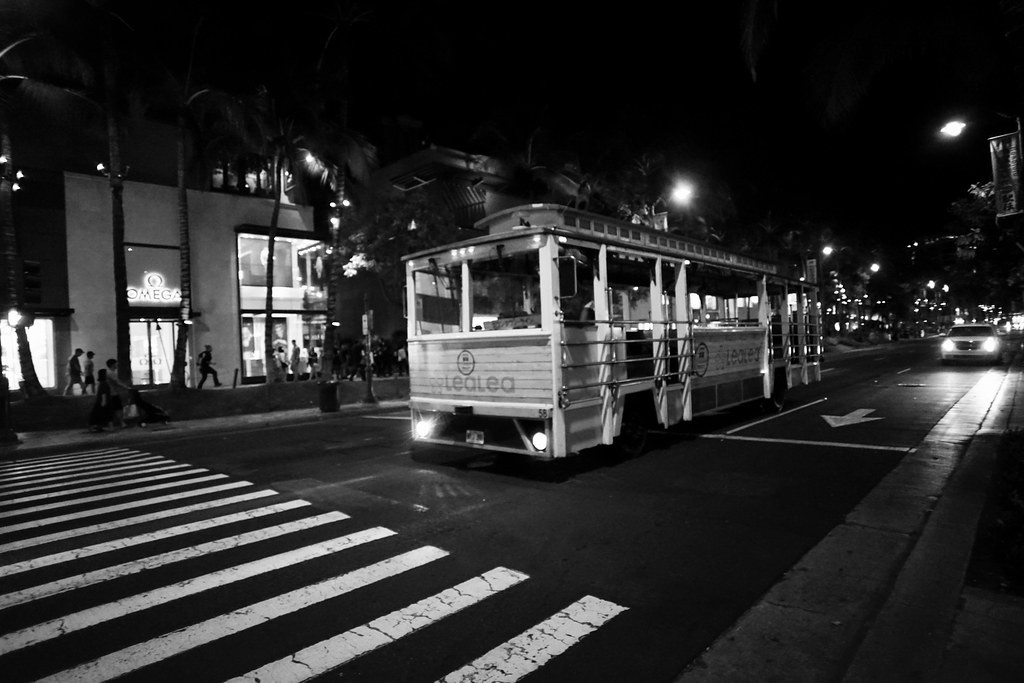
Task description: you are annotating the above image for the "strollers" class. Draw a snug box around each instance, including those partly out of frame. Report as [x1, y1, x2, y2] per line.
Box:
[129, 388, 172, 430]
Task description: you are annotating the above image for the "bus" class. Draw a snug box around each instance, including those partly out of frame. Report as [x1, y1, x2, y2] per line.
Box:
[399, 204, 822, 466]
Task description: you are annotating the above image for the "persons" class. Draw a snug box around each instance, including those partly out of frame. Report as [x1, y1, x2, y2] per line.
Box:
[273, 346, 289, 383]
[196, 345, 222, 389]
[272, 324, 287, 348]
[290, 341, 300, 383]
[398, 345, 409, 376]
[306, 346, 318, 380]
[62, 348, 87, 395]
[88, 359, 130, 431]
[333, 338, 392, 382]
[81, 351, 96, 394]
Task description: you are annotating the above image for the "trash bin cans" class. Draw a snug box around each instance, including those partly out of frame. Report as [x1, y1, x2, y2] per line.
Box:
[316, 380, 342, 413]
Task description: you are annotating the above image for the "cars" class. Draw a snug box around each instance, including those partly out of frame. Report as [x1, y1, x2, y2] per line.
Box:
[941, 322, 1004, 366]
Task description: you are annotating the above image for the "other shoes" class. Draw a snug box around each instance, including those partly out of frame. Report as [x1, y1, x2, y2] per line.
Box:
[97, 428, 105, 431]
[120, 424, 128, 429]
[215, 383, 222, 386]
[198, 386, 202, 390]
